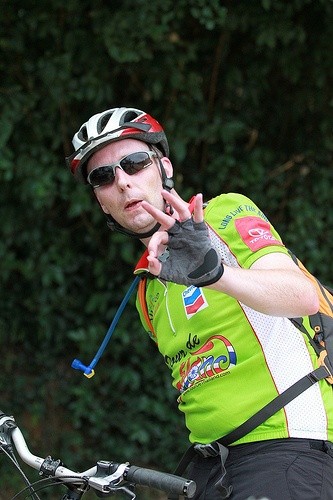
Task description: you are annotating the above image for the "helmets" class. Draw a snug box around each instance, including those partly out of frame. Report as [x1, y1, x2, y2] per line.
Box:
[65, 108, 169, 177]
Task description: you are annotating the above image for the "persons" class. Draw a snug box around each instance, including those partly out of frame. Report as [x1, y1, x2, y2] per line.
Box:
[67, 107, 333, 500]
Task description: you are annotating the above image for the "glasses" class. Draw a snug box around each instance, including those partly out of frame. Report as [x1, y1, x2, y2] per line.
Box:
[85, 151, 160, 190]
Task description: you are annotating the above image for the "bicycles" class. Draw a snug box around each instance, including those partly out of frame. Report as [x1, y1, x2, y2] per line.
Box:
[0, 411, 197, 500]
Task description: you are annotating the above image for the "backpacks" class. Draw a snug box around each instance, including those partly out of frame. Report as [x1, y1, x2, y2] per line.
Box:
[137, 193, 333, 458]
